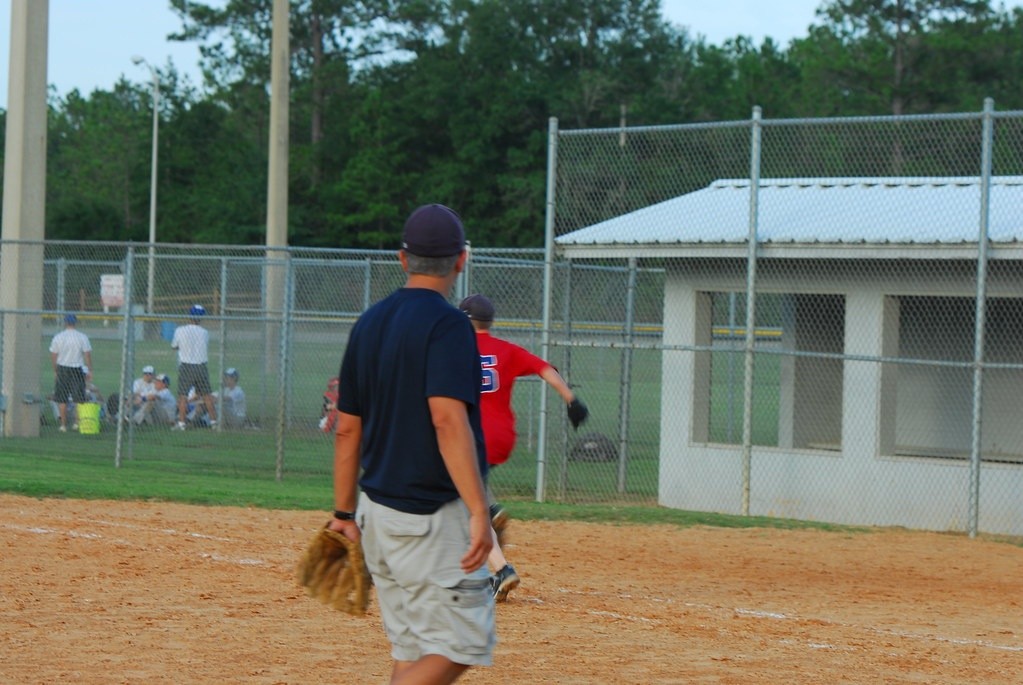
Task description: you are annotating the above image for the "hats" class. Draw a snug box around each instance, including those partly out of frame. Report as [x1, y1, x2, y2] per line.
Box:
[189, 304, 206, 316]
[157, 374, 170, 385]
[224, 367, 239, 382]
[456, 294, 495, 321]
[143, 365, 154, 374]
[64, 314, 77, 324]
[401, 203, 465, 258]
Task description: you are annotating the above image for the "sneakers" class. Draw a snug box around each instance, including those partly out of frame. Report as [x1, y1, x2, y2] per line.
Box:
[489, 565, 520, 603]
[59, 426, 67, 432]
[171, 420, 186, 433]
[209, 420, 218, 431]
[489, 502, 509, 531]
[72, 424, 79, 431]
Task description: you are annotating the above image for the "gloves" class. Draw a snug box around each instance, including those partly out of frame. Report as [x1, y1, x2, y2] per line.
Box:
[566, 398, 588, 429]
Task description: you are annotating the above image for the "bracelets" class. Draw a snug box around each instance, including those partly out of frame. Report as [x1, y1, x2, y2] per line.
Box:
[334, 509, 356, 521]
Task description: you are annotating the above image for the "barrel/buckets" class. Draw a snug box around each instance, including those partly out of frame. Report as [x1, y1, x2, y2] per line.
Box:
[75, 402, 101, 435]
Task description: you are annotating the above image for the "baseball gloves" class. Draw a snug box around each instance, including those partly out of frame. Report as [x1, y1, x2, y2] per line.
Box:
[292, 518, 375, 621]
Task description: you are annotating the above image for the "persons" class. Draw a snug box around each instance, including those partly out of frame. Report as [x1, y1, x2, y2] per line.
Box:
[49, 314, 93, 432]
[172, 305, 218, 430]
[459, 295, 588, 602]
[50, 364, 247, 429]
[325, 204, 497, 685]
